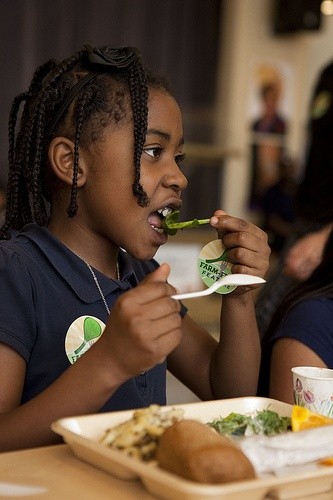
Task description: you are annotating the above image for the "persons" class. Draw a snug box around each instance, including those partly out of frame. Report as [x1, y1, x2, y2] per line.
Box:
[264, 56, 333, 404]
[247, 82, 285, 199]
[0, 46, 271, 454]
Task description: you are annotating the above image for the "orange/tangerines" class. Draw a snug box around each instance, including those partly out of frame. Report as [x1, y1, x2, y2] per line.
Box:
[291, 404, 333, 467]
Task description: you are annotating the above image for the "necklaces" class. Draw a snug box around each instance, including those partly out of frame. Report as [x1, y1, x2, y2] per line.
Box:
[77, 255, 120, 316]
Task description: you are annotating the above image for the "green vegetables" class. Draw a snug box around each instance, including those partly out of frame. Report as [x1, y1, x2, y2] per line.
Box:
[206, 408, 294, 437]
[161, 210, 211, 236]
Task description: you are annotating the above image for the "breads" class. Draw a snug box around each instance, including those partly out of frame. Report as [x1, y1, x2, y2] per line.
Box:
[157, 419, 256, 484]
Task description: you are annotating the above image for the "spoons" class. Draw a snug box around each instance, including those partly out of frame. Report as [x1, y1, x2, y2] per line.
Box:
[171, 274, 267, 300]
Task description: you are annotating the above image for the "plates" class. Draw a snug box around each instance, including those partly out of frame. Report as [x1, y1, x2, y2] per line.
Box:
[51, 396, 333, 500]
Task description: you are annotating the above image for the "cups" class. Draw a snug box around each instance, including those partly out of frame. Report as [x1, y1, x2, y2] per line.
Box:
[291, 366, 333, 418]
